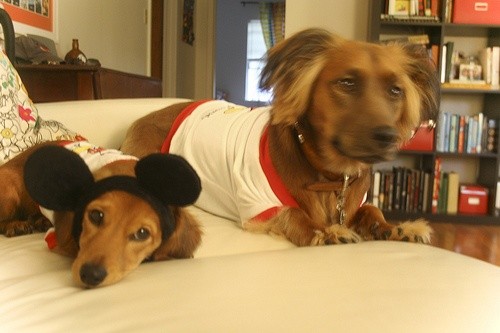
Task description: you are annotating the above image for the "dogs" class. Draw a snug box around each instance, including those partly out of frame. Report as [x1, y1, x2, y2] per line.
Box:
[0, 139, 203, 291]
[119, 28, 440, 246]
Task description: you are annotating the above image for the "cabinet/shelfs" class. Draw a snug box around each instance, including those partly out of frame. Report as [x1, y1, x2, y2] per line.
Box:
[367, 0, 500, 266]
[12, 62, 163, 105]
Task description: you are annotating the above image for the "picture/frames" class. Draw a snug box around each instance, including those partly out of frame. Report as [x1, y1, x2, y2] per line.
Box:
[0, 0, 59, 42]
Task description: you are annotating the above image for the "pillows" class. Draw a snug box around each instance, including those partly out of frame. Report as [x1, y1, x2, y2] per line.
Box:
[0, 48, 88, 169]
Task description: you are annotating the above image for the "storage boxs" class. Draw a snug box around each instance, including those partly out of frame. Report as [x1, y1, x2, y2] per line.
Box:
[399, 120, 435, 151]
[451, 0, 500, 26]
[458, 184, 489, 214]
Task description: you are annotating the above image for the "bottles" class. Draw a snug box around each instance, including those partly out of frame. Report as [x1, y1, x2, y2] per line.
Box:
[63, 38, 87, 65]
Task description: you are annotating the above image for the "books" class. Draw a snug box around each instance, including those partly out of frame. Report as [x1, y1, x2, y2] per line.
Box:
[385, 0, 442, 16]
[378, 34, 500, 84]
[373, 166, 459, 216]
[437, 110, 496, 153]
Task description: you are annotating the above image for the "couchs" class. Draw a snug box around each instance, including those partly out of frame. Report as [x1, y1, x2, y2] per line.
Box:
[0, 97, 500, 333]
